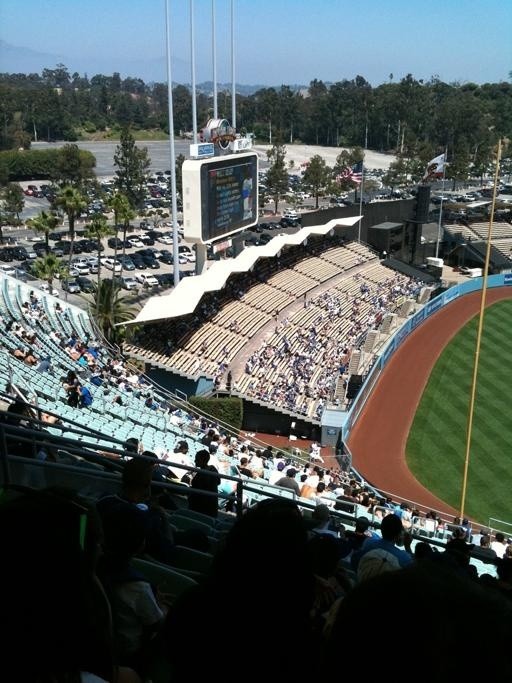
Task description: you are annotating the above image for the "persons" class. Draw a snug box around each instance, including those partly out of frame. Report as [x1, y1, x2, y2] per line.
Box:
[161, 499, 317, 683]
[1, 257, 511, 662]
[0, 488, 142, 683]
[318, 565, 512, 681]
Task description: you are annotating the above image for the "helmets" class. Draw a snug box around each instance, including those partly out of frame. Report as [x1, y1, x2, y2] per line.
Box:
[287, 468, 299, 475]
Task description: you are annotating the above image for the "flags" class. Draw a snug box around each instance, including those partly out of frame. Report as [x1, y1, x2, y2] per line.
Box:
[421, 153, 444, 182]
[334, 160, 362, 185]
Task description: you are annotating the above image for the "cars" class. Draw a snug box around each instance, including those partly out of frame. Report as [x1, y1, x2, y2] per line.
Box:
[246, 160, 425, 245]
[427, 162, 512, 204]
[192, 243, 217, 259]
[0, 169, 196, 294]
[227, 247, 234, 256]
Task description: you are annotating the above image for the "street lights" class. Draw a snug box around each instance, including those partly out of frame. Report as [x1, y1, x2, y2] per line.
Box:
[435, 161, 450, 258]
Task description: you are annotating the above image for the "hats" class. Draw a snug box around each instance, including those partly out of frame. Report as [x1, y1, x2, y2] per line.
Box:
[493, 557, 512, 571]
[446, 538, 476, 551]
